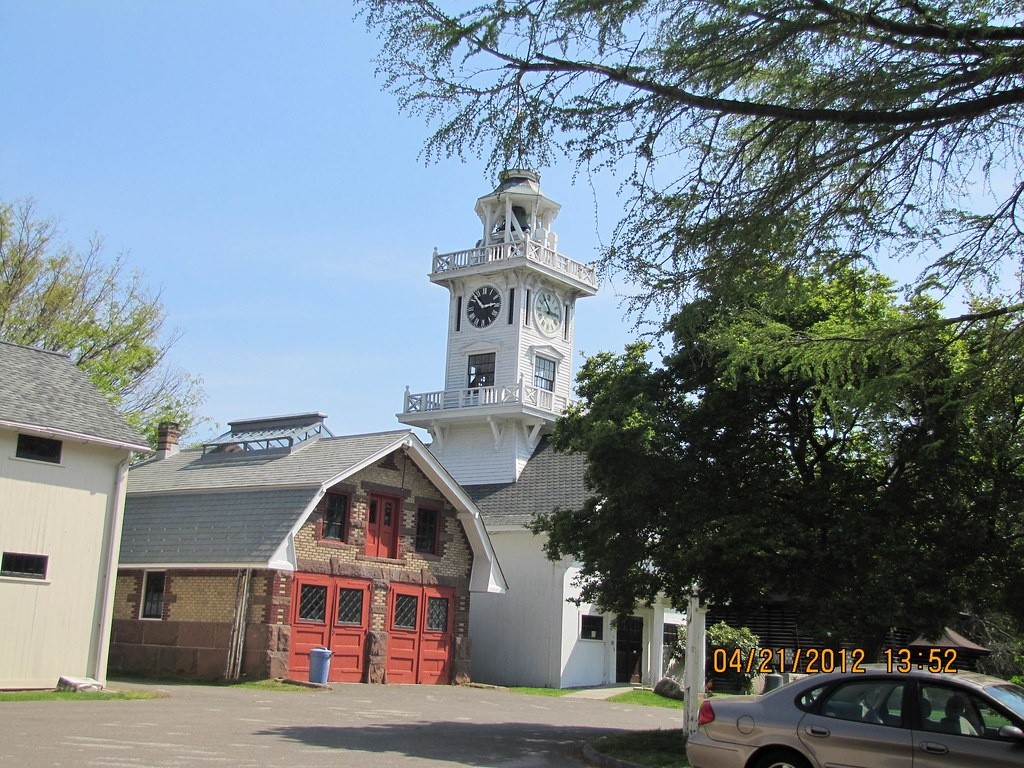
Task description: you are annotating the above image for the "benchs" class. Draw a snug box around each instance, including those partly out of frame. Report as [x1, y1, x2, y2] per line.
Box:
[804, 701, 862, 720]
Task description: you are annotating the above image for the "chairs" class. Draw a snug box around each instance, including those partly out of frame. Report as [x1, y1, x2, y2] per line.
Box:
[921, 697, 936, 731]
[939, 695, 979, 736]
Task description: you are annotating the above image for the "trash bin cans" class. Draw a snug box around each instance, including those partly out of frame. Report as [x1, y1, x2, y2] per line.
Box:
[308, 649, 332, 683]
[764, 674, 784, 695]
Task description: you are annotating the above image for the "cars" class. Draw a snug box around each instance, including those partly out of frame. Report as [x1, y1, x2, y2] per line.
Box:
[686, 663, 1024, 768]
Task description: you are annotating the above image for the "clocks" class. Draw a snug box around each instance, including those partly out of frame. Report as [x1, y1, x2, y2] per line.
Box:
[465, 282, 504, 332]
[533, 287, 565, 337]
